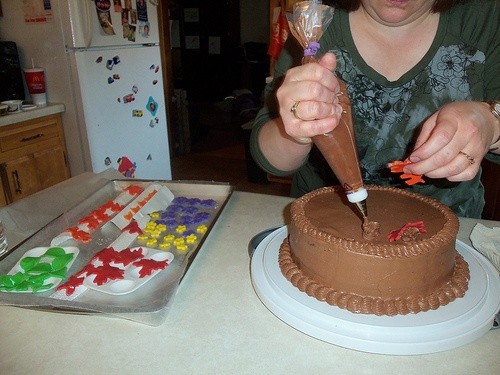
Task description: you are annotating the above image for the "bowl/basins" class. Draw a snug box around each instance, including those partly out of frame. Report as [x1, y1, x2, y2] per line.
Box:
[1, 100, 23, 114]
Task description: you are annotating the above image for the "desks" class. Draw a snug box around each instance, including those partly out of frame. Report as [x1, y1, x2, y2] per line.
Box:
[0, 189, 500, 375]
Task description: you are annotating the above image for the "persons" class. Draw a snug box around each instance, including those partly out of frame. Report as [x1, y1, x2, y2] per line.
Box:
[122, 13, 128, 24]
[136, 0, 146, 19]
[249, 0, 500, 219]
[102, 14, 112, 32]
[141, 25, 149, 38]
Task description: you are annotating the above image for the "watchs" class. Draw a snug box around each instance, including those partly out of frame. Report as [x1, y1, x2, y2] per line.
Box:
[481, 100, 500, 121]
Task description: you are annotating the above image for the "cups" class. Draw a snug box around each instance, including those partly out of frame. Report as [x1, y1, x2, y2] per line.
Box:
[23, 68, 46, 105]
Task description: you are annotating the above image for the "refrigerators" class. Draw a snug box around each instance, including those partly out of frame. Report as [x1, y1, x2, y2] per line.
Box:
[0, 0, 172, 180]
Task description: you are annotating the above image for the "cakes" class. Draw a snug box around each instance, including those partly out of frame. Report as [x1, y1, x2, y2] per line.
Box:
[278, 185, 470, 315]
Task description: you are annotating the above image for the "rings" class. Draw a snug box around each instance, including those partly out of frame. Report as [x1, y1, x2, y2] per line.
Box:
[291, 100, 300, 120]
[459, 151, 475, 165]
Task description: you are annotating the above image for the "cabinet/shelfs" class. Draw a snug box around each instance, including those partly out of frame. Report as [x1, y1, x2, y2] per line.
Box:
[0, 111, 72, 208]
